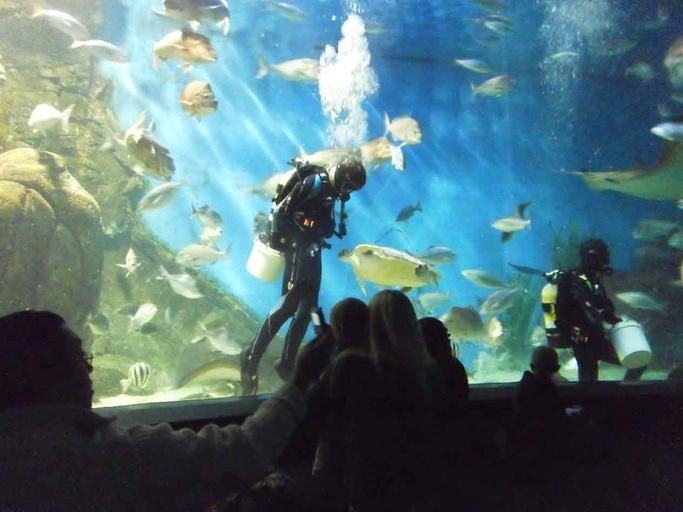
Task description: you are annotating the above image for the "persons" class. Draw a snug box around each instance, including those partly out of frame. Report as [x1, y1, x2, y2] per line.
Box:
[0, 310, 333, 512]
[301, 297, 381, 483]
[354, 291, 436, 475]
[413, 317, 468, 417]
[235, 156, 369, 389]
[558, 238, 655, 382]
[514, 345, 564, 430]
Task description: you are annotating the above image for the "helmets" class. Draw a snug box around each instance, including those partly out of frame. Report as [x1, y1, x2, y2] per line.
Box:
[580, 237, 609, 270]
[335, 160, 366, 194]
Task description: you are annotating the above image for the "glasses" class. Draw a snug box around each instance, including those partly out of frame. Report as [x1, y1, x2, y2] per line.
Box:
[55, 351, 94, 367]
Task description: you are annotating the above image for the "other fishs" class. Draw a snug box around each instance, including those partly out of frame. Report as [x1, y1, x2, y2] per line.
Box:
[27, 0, 683, 401]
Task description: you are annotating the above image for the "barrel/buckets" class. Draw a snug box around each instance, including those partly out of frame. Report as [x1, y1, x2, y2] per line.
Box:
[611, 316, 653, 371]
[246, 233, 285, 282]
